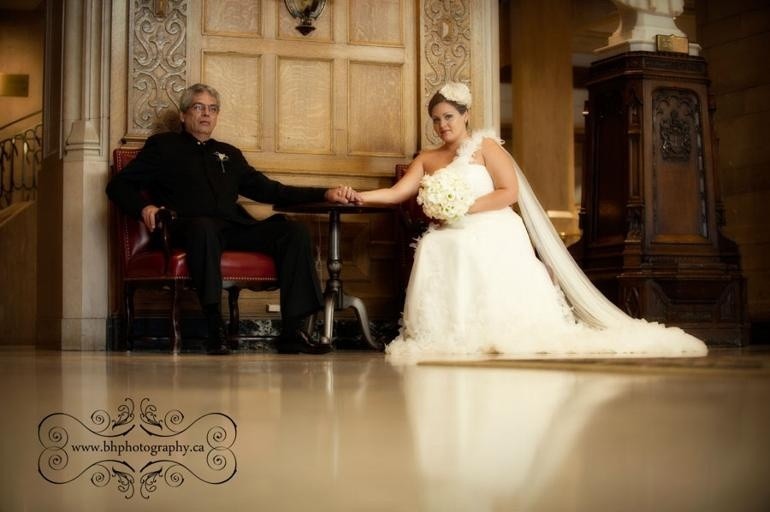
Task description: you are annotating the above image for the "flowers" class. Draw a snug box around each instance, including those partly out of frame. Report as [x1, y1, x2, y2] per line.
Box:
[416, 168, 475, 225]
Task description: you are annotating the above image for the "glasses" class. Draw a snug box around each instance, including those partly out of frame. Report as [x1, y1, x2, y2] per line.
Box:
[189, 103, 219, 114]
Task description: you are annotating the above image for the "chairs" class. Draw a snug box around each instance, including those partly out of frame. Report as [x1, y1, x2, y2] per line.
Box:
[393, 164, 433, 333]
[110, 147, 282, 356]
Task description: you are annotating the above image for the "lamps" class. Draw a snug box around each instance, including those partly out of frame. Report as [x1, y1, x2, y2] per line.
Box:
[284, 0, 326, 35]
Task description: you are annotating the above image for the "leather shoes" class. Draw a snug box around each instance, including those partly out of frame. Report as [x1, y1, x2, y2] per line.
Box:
[279, 328, 333, 355]
[203, 326, 230, 354]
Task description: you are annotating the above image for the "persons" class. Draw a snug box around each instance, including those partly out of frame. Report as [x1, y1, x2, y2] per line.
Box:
[336, 81, 579, 355]
[104, 83, 364, 357]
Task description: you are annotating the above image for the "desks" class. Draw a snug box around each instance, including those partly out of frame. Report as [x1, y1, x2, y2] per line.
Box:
[271, 203, 390, 352]
